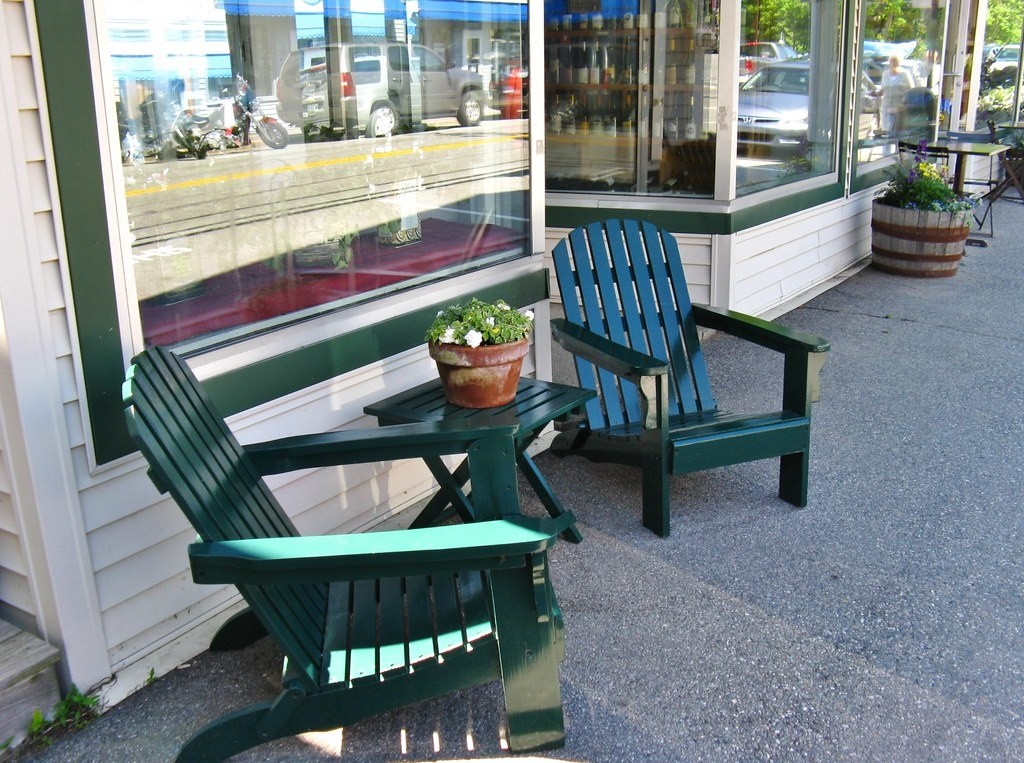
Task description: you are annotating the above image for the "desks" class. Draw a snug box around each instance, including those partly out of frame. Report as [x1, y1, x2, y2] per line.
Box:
[903, 138, 1012, 239]
[363, 376, 597, 544]
[544, 26, 701, 172]
[997, 121, 1024, 130]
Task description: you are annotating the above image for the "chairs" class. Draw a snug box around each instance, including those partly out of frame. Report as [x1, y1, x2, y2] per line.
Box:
[550, 218, 831, 539]
[980, 120, 1024, 203]
[945, 132, 996, 238]
[122, 346, 577, 763]
[898, 139, 982, 227]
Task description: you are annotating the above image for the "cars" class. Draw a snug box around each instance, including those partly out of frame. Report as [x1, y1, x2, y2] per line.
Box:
[983, 43, 1021, 82]
[488, 53, 531, 114]
[737, 62, 812, 157]
[863, 57, 921, 83]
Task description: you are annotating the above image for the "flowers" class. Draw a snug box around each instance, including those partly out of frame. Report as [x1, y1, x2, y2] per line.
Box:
[872, 136, 984, 229]
[423, 299, 535, 346]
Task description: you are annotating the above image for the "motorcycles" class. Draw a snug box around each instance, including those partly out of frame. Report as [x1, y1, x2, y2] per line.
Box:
[116, 75, 289, 159]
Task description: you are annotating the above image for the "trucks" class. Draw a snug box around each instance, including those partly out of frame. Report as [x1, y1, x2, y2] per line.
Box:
[739, 41, 796, 81]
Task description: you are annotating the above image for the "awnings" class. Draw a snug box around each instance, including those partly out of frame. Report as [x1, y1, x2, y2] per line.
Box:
[297, 16, 415, 38]
[114, 54, 232, 79]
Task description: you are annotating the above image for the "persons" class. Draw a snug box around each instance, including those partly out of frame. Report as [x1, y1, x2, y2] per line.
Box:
[914, 50, 939, 87]
[868, 56, 912, 154]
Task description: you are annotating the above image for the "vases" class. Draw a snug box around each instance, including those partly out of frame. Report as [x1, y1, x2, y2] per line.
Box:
[427, 336, 534, 409]
[871, 197, 973, 279]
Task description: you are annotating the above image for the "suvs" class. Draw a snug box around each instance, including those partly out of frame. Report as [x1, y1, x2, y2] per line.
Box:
[275, 42, 485, 138]
[306, 54, 397, 138]
